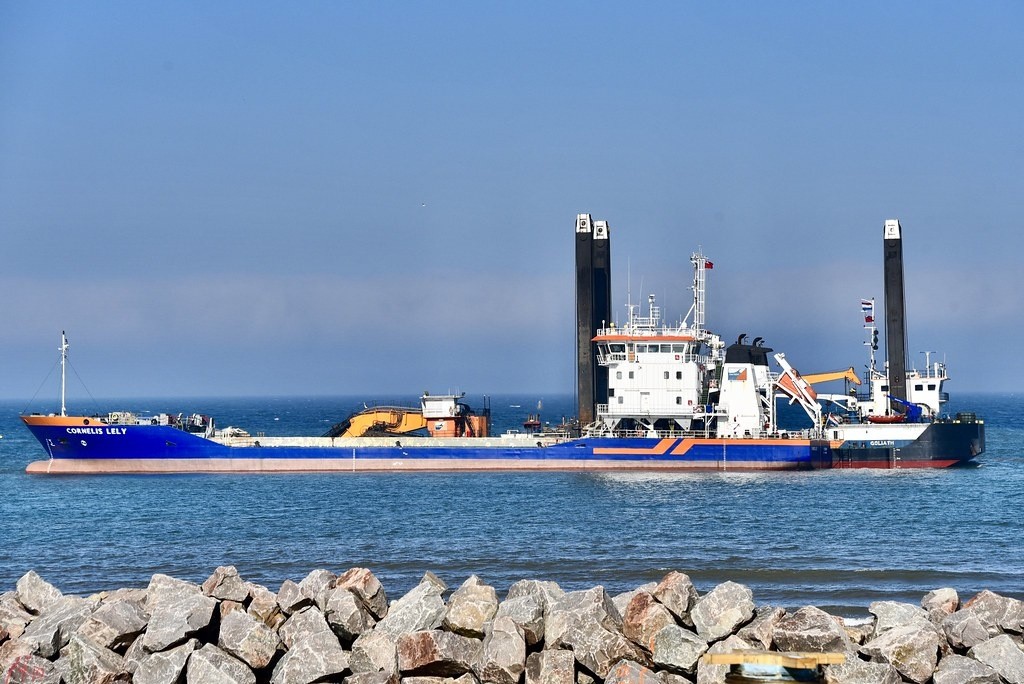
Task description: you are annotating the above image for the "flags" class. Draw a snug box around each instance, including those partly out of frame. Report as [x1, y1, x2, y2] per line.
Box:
[705, 261, 713, 269]
[866, 315, 873, 323]
[861, 300, 872, 310]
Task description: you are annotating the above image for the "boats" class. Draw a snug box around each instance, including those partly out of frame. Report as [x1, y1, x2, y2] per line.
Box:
[313, 217, 988, 470]
[18, 210, 833, 476]
